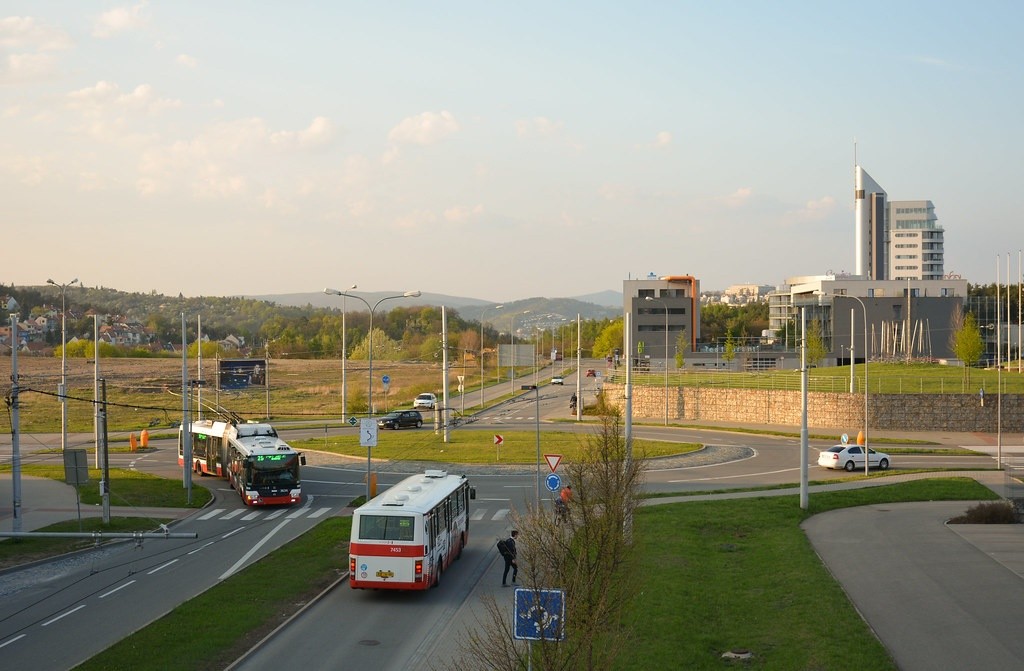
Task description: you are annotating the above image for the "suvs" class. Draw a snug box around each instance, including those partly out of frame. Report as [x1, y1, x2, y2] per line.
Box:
[551, 375, 563, 385]
[377, 410, 422, 430]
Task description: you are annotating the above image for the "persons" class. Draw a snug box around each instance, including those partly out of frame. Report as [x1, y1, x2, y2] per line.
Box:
[249, 364, 265, 385]
[280, 468, 294, 480]
[569, 392, 577, 408]
[561, 485, 572, 523]
[501, 530, 519, 587]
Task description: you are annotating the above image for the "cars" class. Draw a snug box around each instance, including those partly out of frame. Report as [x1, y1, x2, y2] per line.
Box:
[414, 393, 437, 410]
[586, 369, 596, 377]
[818, 444, 891, 471]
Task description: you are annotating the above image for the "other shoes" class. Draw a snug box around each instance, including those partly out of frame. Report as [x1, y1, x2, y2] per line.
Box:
[502, 582, 519, 587]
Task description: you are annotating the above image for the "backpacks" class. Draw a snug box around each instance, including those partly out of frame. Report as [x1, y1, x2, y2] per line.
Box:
[497, 540, 516, 566]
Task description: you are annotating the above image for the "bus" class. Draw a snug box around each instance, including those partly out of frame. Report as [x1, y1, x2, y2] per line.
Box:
[633, 358, 651, 372]
[550, 348, 562, 360]
[349, 469, 475, 590]
[178, 417, 306, 506]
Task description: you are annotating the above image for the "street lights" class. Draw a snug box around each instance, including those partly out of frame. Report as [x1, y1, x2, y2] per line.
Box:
[512, 310, 530, 396]
[813, 291, 869, 476]
[481, 305, 504, 408]
[562, 320, 575, 389]
[536, 315, 552, 385]
[645, 296, 668, 426]
[47, 279, 79, 451]
[324, 288, 421, 501]
